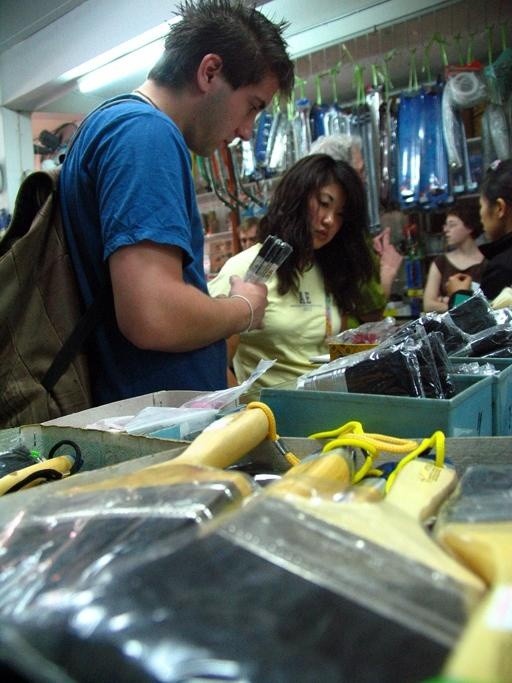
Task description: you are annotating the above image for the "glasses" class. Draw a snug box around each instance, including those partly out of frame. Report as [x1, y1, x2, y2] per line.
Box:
[486, 158, 503, 177]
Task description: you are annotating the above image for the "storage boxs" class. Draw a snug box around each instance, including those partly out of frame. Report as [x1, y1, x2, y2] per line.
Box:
[0, 339, 512, 528]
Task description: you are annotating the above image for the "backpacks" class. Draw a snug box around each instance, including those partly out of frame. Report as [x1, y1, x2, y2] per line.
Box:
[0, 92, 150, 430]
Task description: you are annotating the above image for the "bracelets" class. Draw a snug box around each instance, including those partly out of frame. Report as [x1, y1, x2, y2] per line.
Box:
[230, 292, 254, 336]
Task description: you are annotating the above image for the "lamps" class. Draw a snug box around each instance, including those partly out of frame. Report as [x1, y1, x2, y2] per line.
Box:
[75, 36, 169, 95]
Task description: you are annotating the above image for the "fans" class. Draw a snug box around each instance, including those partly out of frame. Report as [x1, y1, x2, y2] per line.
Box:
[33, 123, 79, 167]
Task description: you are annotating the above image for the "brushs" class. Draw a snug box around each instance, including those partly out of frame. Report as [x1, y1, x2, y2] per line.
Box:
[1, 400, 395, 682]
[295, 288, 512, 399]
[28, 431, 481, 682]
[434, 460, 512, 683]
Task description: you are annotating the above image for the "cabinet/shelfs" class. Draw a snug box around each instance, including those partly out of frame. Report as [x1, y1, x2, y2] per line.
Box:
[194, 69, 512, 300]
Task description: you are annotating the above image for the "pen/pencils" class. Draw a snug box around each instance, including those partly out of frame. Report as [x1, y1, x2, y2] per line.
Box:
[244, 234, 293, 284]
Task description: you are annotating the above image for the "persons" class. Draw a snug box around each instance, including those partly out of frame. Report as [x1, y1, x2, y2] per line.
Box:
[306, 133, 403, 331]
[420, 203, 483, 314]
[206, 150, 373, 407]
[56, 0, 297, 411]
[444, 155, 510, 309]
